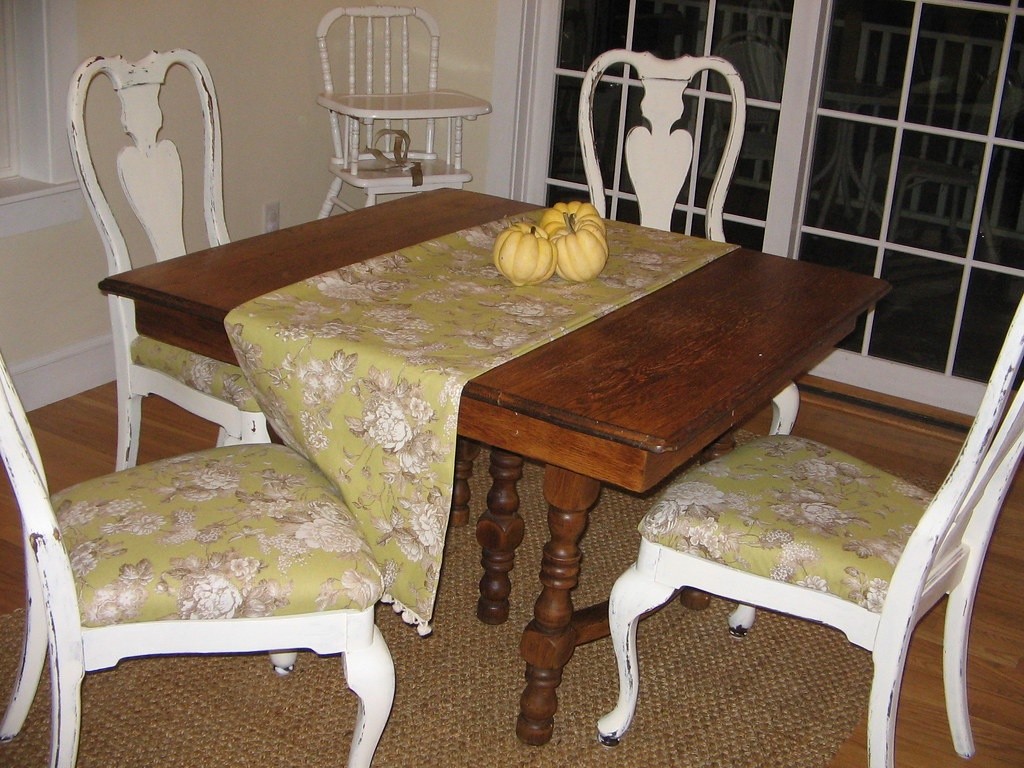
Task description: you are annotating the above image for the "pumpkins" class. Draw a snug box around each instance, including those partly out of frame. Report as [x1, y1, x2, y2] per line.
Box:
[540, 202, 599, 234]
[549, 212, 609, 283]
[492, 222, 558, 286]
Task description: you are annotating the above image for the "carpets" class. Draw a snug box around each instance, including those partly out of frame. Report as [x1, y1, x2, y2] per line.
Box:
[0, 446, 875, 768]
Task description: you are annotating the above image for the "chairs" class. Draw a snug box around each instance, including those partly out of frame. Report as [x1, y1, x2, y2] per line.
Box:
[857, 67, 1022, 265]
[0, 350, 396, 768]
[597, 292, 1024, 767]
[696, 30, 786, 190]
[67, 46, 274, 474]
[577, 48, 800, 434]
[316, 6, 492, 220]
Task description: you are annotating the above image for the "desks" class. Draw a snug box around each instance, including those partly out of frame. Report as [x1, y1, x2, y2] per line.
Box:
[97, 186, 894, 747]
[809, 78, 916, 240]
[317, 88, 493, 125]
